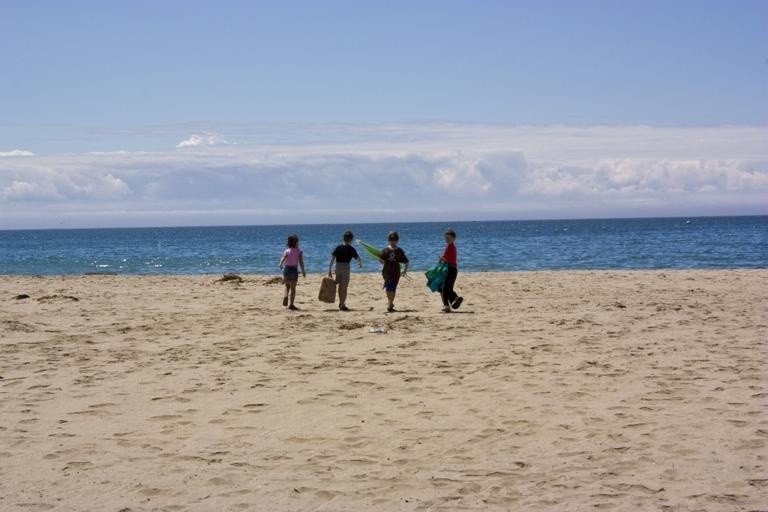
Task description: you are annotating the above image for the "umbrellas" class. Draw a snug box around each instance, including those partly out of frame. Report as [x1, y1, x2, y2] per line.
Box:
[356, 239, 411, 281]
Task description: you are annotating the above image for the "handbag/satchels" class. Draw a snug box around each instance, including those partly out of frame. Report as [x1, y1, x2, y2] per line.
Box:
[318, 275, 336, 302]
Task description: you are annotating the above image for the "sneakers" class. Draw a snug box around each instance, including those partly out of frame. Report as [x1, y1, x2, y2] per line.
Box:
[441, 296, 463, 312]
[283, 296, 298, 310]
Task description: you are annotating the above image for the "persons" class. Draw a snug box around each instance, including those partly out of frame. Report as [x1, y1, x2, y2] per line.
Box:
[439, 229, 463, 312]
[280, 235, 305, 310]
[380, 232, 409, 311]
[328, 231, 362, 311]
